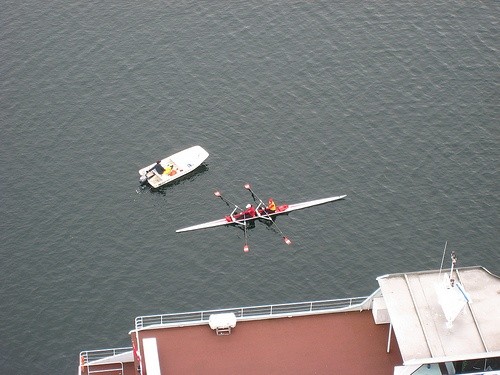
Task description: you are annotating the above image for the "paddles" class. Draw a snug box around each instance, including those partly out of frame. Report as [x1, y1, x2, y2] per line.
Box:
[213, 191, 242, 211]
[245, 184, 266, 207]
[244, 215, 249, 252]
[263, 210, 291, 245]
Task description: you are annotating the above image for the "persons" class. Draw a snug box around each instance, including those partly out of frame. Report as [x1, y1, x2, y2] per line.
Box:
[237, 204, 255, 220]
[146, 159, 173, 174]
[260, 198, 276, 216]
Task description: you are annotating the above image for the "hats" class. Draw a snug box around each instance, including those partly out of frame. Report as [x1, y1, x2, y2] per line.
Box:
[268, 199, 272, 202]
[156, 160, 161, 163]
[246, 204, 251, 208]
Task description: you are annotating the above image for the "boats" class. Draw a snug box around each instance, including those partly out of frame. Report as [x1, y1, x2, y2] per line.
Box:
[78, 240, 500, 375]
[138, 145, 209, 189]
[175, 194, 347, 233]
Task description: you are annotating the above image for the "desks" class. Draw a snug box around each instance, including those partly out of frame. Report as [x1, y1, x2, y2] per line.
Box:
[208, 313, 236, 329]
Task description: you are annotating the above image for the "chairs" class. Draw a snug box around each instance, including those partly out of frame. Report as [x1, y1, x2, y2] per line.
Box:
[216, 326, 232, 336]
